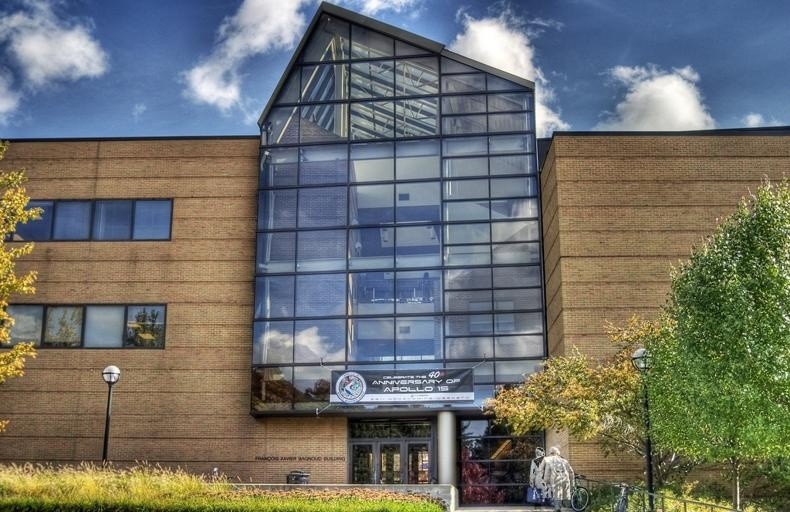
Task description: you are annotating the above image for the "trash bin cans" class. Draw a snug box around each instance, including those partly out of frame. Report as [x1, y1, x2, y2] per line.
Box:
[286, 469, 310, 484]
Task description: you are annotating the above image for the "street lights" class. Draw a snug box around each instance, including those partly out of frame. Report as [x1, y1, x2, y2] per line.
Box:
[630, 348, 655, 512]
[100, 365, 122, 469]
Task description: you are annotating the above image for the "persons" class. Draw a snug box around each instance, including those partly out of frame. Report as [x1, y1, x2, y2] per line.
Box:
[535, 446, 575, 511]
[529, 446, 548, 506]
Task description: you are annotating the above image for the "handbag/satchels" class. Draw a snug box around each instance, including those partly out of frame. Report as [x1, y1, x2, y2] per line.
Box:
[527, 487, 545, 503]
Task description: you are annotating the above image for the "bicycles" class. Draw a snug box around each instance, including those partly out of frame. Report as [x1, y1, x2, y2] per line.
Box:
[562, 473, 590, 511]
[612, 483, 640, 511]
